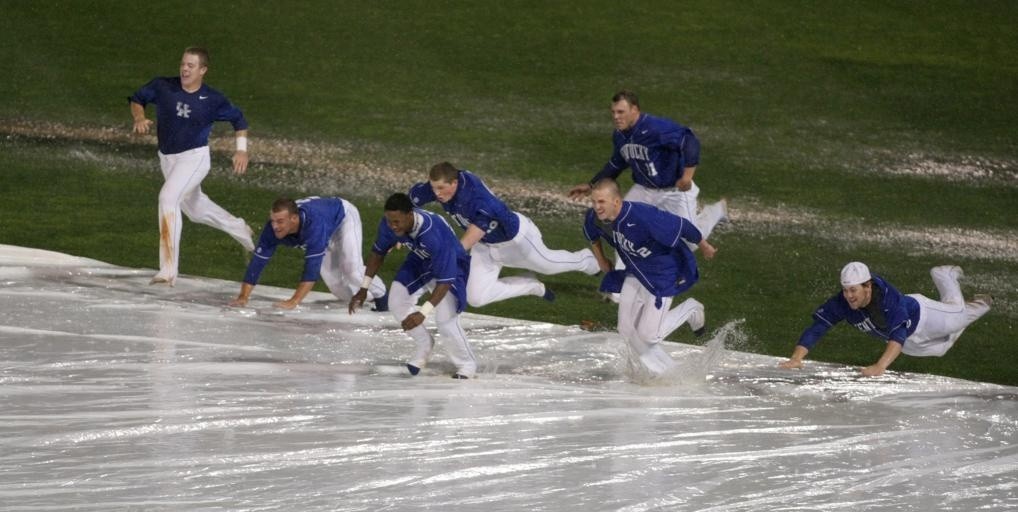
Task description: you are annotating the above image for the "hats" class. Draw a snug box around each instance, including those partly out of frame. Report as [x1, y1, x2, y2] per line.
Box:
[839, 261, 872, 286]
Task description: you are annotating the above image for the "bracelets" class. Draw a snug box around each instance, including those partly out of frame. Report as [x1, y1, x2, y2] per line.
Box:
[360, 276, 372, 289]
[235, 136, 246, 152]
[418, 300, 433, 316]
[288, 297, 297, 306]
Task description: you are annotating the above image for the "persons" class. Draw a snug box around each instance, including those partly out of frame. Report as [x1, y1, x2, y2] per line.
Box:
[396, 163, 602, 308]
[779, 261, 992, 376]
[348, 193, 477, 380]
[229, 196, 389, 311]
[583, 177, 715, 347]
[128, 47, 255, 287]
[569, 91, 728, 304]
